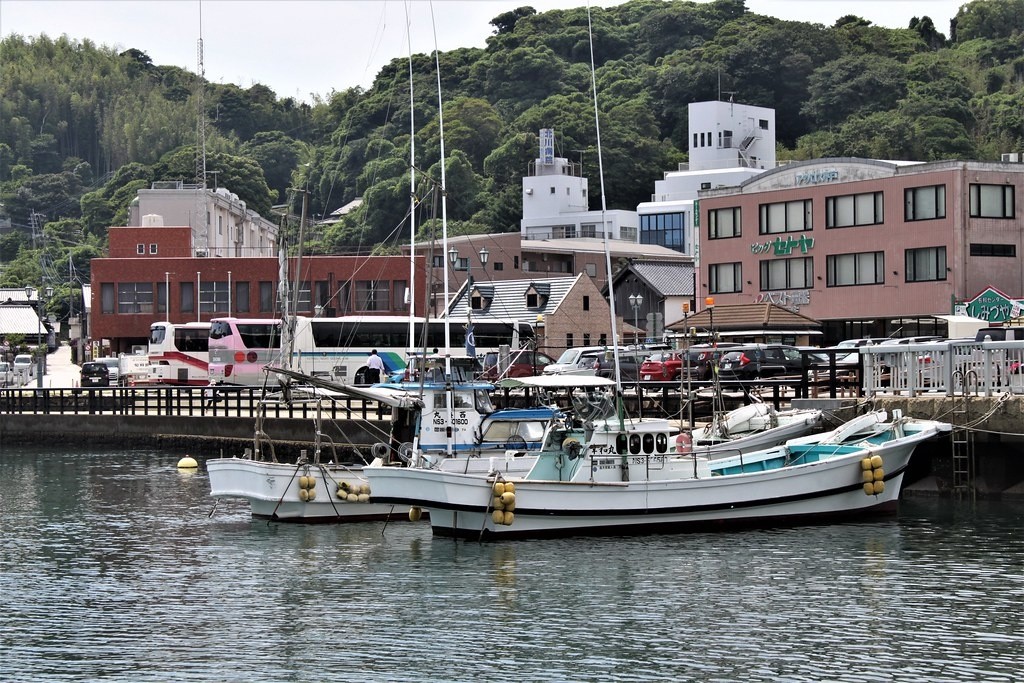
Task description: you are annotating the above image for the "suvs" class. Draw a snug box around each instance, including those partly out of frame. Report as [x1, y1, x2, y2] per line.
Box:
[718, 344, 811, 392]
[675, 343, 744, 390]
[809, 335, 973, 391]
[640, 350, 688, 391]
[578, 350, 648, 383]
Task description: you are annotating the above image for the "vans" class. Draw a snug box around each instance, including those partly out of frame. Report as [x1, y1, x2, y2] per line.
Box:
[482, 350, 557, 382]
[409, 355, 483, 383]
[81, 362, 109, 387]
[13, 354, 34, 376]
[0, 362, 14, 385]
[542, 345, 630, 376]
[94, 359, 118, 386]
[973, 325, 1024, 386]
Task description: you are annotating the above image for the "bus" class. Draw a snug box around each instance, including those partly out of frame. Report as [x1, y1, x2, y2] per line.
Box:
[208, 318, 283, 392]
[278, 315, 537, 387]
[147, 322, 209, 387]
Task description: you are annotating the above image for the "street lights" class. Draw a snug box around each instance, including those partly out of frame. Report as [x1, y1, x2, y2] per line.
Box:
[628, 292, 643, 344]
[448, 243, 489, 320]
[25, 284, 54, 396]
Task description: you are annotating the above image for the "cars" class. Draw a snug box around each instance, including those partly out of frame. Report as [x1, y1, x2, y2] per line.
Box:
[388, 368, 406, 384]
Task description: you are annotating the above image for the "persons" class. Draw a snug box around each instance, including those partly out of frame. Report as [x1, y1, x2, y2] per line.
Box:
[367, 349, 385, 383]
[429, 347, 441, 363]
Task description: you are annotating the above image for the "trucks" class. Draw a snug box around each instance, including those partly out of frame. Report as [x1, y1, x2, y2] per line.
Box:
[118, 352, 148, 388]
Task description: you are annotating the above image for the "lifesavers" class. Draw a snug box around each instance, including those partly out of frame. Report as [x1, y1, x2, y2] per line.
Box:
[676, 434, 692, 455]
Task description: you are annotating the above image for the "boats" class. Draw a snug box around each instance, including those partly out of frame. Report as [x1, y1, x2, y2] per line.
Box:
[205, 0, 827, 523]
[364, 0, 950, 543]
